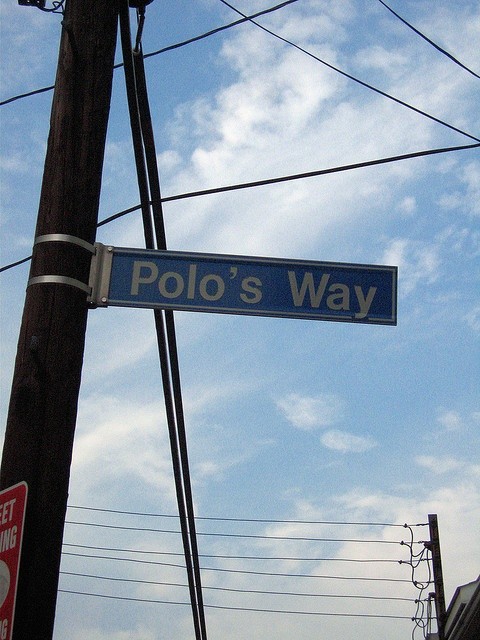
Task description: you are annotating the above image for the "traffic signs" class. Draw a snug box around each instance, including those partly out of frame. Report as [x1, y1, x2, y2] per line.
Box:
[90, 241, 397, 328]
